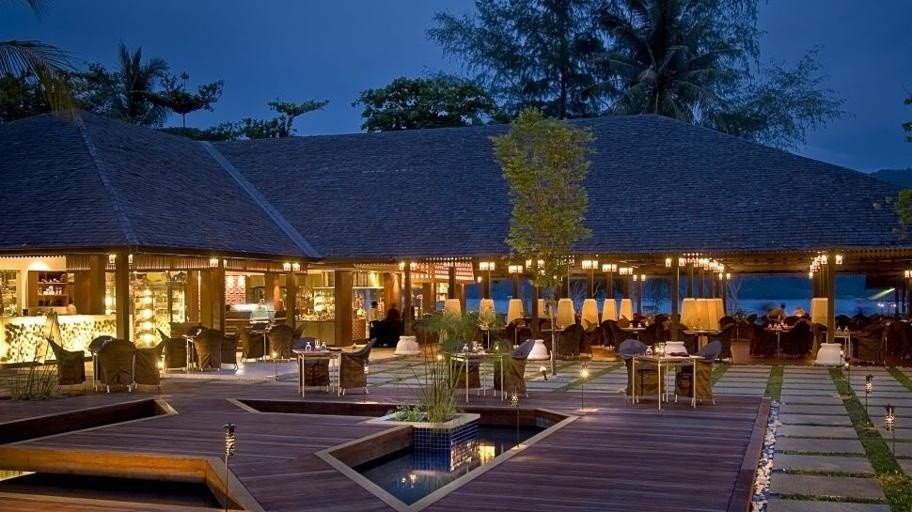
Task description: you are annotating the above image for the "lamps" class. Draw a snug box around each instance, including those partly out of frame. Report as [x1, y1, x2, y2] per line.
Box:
[808, 254, 844, 282]
[400, 261, 417, 271]
[209, 257, 227, 268]
[581, 259, 645, 281]
[477, 260, 496, 282]
[665, 256, 730, 280]
[508, 259, 557, 280]
[283, 262, 300, 272]
[109, 254, 132, 266]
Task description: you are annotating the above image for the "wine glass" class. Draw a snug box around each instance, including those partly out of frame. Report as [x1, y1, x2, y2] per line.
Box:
[304, 341, 327, 353]
[46, 287, 62, 295]
[462, 343, 485, 356]
[645, 346, 653, 356]
[629, 323, 642, 328]
[768, 322, 848, 334]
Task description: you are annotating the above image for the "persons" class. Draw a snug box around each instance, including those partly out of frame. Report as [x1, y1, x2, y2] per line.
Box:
[628, 313, 639, 327]
[767, 304, 786, 322]
[383, 303, 401, 346]
[365, 301, 381, 347]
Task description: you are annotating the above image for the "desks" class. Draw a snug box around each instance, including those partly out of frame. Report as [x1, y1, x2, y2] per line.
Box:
[291, 347, 342, 398]
[715, 310, 912, 365]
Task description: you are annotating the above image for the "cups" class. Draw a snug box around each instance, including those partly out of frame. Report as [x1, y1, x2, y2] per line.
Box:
[321, 313, 327, 321]
[659, 342, 666, 356]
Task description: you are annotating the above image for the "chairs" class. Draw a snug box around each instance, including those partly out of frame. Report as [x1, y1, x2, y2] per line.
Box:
[156, 326, 243, 372]
[298, 337, 377, 396]
[45, 336, 87, 392]
[444, 338, 535, 401]
[88, 335, 167, 392]
[451, 318, 697, 360]
[620, 337, 722, 409]
[239, 322, 305, 365]
